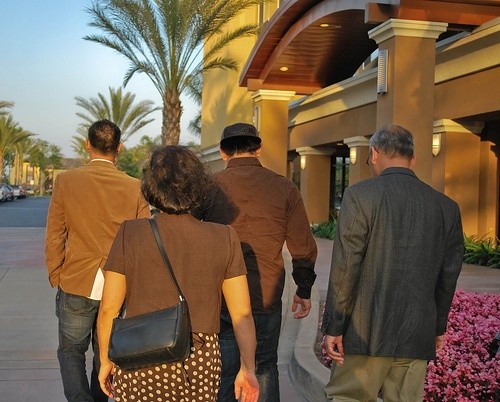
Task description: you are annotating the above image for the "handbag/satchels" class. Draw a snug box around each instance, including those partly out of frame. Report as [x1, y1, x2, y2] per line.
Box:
[107, 301, 193, 369]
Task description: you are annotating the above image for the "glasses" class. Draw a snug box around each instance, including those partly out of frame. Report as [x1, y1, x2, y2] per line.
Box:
[366, 145, 379, 164]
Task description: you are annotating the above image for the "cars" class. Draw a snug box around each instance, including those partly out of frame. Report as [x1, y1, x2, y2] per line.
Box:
[0, 182, 35, 203]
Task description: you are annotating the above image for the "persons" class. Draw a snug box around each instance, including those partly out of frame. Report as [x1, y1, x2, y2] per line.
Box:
[97, 144, 260, 402]
[45, 119, 152, 402]
[199, 123, 317, 402]
[320, 125, 464, 402]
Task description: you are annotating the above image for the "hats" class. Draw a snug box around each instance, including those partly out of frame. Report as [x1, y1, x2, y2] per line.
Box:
[220, 123, 262, 147]
